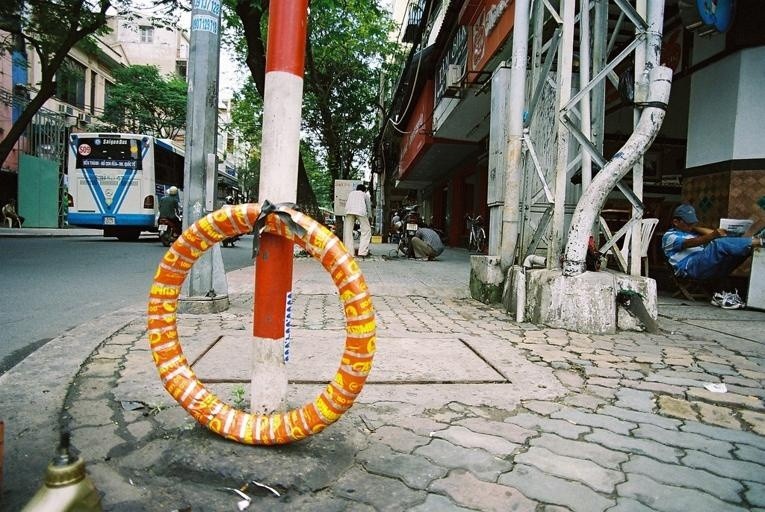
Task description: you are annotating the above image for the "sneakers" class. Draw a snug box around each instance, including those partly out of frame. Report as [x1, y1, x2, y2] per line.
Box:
[415, 257, 429, 262]
[711, 287, 746, 309]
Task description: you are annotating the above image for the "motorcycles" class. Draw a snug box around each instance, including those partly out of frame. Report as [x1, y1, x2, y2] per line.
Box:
[156, 207, 184, 247]
[400, 201, 424, 259]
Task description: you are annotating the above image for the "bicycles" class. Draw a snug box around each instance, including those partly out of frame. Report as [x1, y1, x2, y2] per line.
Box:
[463, 212, 488, 255]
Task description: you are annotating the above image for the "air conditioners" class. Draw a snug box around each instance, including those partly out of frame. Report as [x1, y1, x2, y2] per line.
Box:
[59, 103, 73, 116]
[78, 113, 91, 125]
[444, 64, 463, 90]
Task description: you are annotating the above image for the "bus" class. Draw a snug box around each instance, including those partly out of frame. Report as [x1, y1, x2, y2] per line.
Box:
[64, 130, 245, 243]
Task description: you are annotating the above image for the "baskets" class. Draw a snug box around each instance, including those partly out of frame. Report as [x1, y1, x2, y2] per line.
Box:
[467, 219, 479, 229]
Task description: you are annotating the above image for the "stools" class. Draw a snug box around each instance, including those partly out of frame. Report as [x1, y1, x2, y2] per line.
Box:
[672, 276, 710, 304]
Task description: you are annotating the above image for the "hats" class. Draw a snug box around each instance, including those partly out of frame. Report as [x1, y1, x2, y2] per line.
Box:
[673, 204, 700, 224]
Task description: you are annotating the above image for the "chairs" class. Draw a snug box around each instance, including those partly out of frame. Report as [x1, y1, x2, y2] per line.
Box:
[1, 206, 13, 228]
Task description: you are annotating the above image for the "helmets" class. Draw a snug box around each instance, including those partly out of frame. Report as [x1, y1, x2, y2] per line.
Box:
[225, 196, 235, 204]
[169, 186, 178, 196]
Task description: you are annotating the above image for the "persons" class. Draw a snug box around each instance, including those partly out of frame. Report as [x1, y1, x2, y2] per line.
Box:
[4, 198, 25, 228]
[156, 185, 181, 238]
[411, 222, 446, 261]
[222, 193, 257, 247]
[661, 203, 765, 282]
[341, 183, 375, 257]
[390, 211, 400, 232]
